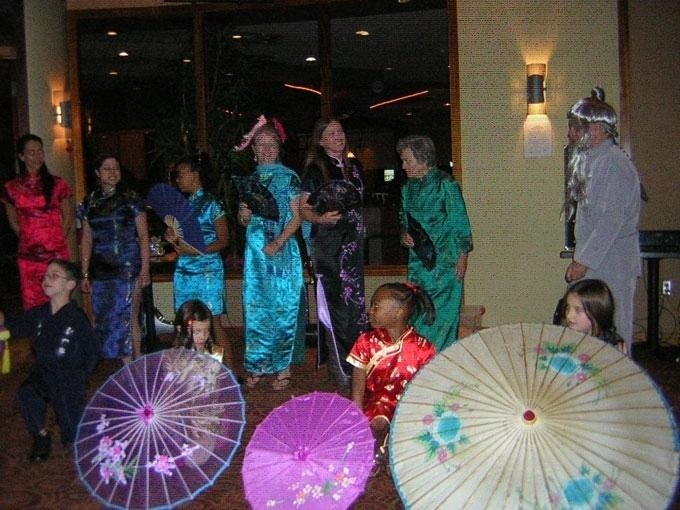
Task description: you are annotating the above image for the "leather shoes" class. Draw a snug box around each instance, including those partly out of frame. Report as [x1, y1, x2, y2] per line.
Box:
[33, 430, 50, 462]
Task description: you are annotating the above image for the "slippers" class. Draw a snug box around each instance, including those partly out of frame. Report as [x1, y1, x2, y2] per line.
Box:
[271, 377, 293, 389]
[247, 372, 265, 386]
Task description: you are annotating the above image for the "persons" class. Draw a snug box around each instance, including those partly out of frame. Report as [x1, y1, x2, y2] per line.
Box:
[345, 282, 437, 478]
[300, 120, 370, 396]
[0, 259, 103, 462]
[231, 114, 302, 391]
[564, 87, 648, 355]
[0, 134, 75, 313]
[564, 278, 626, 355]
[165, 155, 244, 383]
[397, 135, 472, 351]
[79, 153, 150, 368]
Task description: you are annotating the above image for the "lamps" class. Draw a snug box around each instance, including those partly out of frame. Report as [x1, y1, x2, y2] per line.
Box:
[526, 63, 548, 115]
[52, 90, 74, 142]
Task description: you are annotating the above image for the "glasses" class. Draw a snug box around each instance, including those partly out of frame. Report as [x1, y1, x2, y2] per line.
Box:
[41, 271, 72, 281]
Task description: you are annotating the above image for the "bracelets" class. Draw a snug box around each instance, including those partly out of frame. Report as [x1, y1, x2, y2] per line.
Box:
[80, 272, 89, 278]
[240, 218, 248, 226]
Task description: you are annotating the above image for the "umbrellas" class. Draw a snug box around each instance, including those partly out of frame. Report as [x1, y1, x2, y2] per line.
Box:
[74, 346, 245, 510]
[392, 323, 679, 509]
[242, 393, 376, 510]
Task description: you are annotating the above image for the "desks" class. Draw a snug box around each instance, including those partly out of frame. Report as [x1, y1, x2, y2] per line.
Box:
[638, 230, 680, 361]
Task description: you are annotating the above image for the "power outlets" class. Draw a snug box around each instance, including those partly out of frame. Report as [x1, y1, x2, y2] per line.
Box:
[661, 280, 671, 296]
